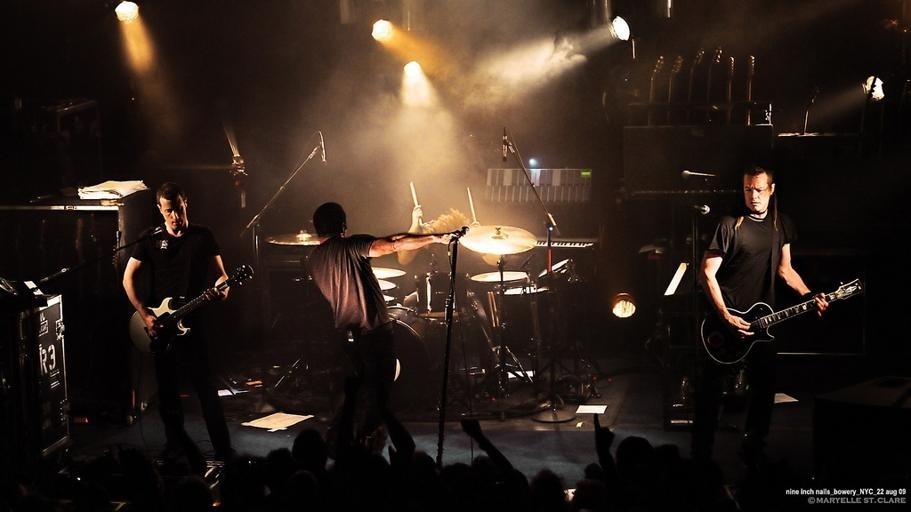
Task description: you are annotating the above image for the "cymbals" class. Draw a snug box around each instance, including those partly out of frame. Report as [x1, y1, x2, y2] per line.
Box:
[378, 279, 398, 291]
[266, 230, 330, 246]
[368, 266, 406, 280]
[459, 223, 537, 254]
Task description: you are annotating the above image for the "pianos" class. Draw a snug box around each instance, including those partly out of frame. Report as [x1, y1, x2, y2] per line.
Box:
[534, 237, 599, 250]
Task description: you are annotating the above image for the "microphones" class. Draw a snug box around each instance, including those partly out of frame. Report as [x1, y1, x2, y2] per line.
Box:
[320, 140, 328, 168]
[502, 133, 508, 162]
[153, 227, 168, 249]
[684, 203, 712, 216]
[679, 169, 717, 180]
[453, 224, 468, 241]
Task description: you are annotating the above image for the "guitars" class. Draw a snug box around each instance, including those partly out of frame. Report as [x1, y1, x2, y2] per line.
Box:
[131, 266, 254, 360]
[702, 277, 868, 366]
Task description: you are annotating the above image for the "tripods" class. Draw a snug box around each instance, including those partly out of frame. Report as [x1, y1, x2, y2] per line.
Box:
[472, 227, 592, 420]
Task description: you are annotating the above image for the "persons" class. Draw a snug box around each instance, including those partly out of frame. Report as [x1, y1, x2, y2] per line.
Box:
[121, 182, 232, 462]
[306, 202, 462, 417]
[688, 164, 830, 459]
[0, 408, 850, 512]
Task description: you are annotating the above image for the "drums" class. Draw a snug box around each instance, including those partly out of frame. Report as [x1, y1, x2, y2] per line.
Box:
[386, 305, 442, 409]
[496, 286, 549, 339]
[469, 271, 536, 320]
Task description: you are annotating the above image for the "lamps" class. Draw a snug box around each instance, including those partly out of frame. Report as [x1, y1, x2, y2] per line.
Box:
[610, 0, 642, 43]
[111, 1, 141, 23]
[368, 13, 401, 46]
[866, 71, 901, 104]
